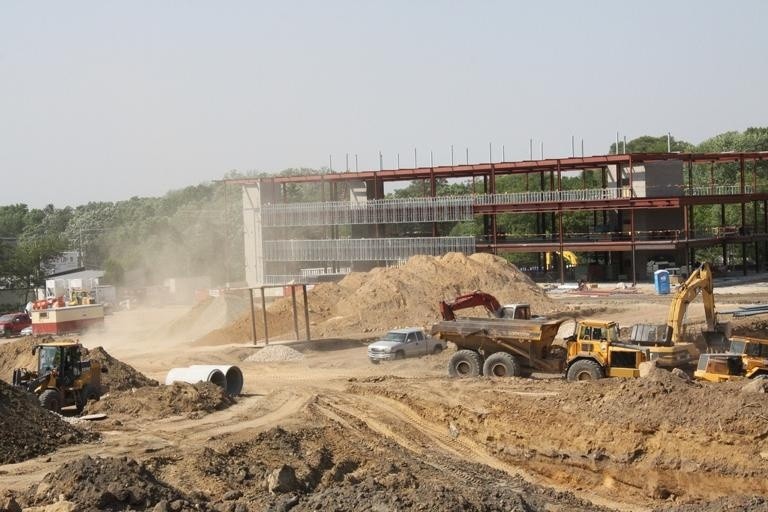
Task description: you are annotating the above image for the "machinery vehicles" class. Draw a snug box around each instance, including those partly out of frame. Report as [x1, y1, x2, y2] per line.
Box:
[13, 342, 102, 414]
[68, 287, 96, 305]
[545, 251, 578, 271]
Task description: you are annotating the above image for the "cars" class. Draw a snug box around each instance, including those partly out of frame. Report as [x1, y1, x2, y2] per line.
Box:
[0, 313, 31, 337]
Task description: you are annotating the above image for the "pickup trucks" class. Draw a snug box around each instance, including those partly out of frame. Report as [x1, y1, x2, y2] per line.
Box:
[367, 328, 447, 364]
